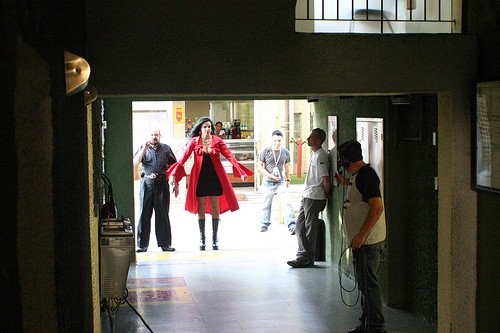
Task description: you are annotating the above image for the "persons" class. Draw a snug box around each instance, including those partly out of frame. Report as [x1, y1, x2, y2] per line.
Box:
[334, 140, 387, 333]
[258, 130, 296, 235]
[166, 117, 253, 251]
[213, 122, 226, 139]
[133, 129, 178, 252]
[287, 128, 330, 268]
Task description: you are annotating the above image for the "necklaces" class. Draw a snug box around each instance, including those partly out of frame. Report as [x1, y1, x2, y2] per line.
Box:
[202, 137, 212, 146]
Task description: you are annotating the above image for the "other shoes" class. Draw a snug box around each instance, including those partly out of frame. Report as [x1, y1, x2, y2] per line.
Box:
[287, 258, 312, 268]
[162, 246, 175, 251]
[347, 326, 361, 333]
[290, 225, 296, 235]
[137, 247, 147, 252]
[259, 225, 267, 232]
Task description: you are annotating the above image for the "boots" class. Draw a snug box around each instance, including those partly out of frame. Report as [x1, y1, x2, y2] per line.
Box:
[198, 219, 205, 250]
[212, 219, 220, 250]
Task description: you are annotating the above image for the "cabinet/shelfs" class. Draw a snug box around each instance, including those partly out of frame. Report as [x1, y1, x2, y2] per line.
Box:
[185, 137, 255, 187]
